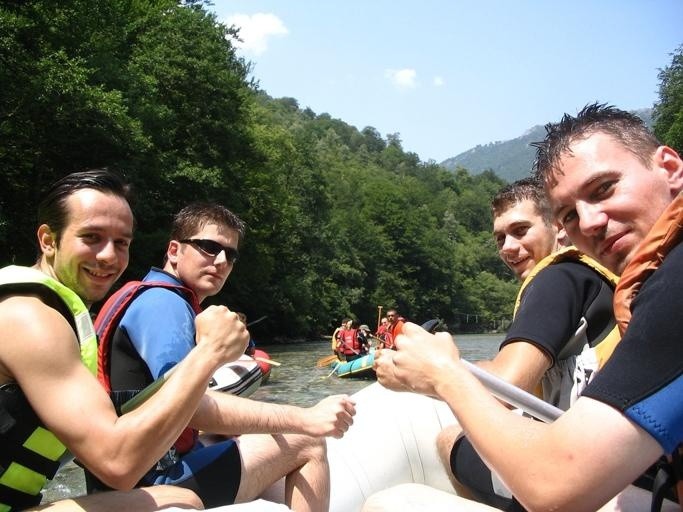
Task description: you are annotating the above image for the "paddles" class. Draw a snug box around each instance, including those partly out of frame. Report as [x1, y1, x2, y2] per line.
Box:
[319, 355, 338, 368]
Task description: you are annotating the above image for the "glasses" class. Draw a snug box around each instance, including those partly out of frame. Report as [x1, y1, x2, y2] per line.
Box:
[177, 238, 240, 265]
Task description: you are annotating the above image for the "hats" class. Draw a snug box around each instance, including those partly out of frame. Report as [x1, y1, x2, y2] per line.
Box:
[358, 324, 371, 332]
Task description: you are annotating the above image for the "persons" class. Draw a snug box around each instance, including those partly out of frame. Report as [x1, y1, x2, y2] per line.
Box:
[392, 176, 627, 511]
[74, 202, 358, 512]
[236, 311, 256, 356]
[331, 307, 404, 363]
[1, 166, 249, 512]
[371, 100, 683, 511]
